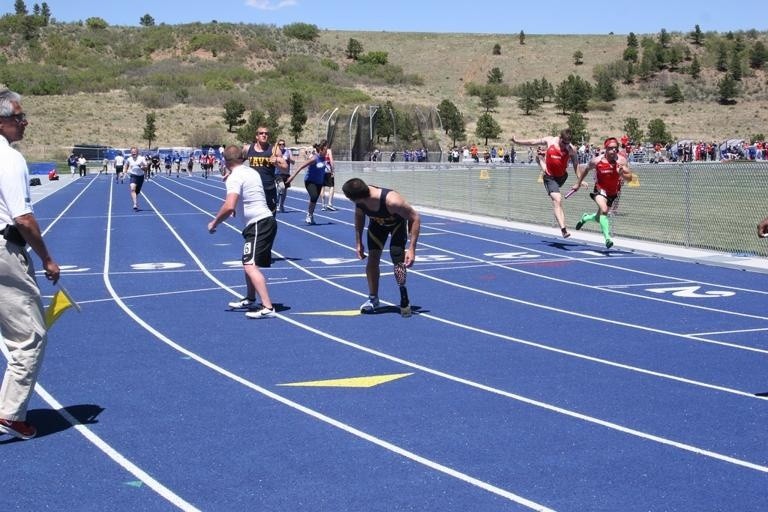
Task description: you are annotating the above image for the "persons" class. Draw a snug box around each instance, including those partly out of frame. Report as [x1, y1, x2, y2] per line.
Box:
[208, 146, 278, 318]
[342, 178, 421, 318]
[758, 216, 768, 238]
[67, 126, 336, 223]
[570, 136, 633, 247]
[0, 85, 61, 442]
[511, 129, 589, 239]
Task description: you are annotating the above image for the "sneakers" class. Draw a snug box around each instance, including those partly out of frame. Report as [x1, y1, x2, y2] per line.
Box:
[319, 205, 327, 211]
[326, 204, 337, 211]
[561, 227, 571, 239]
[226, 296, 258, 312]
[133, 204, 138, 210]
[575, 212, 589, 231]
[399, 303, 412, 318]
[359, 294, 380, 314]
[274, 202, 285, 213]
[0, 416, 40, 440]
[605, 239, 614, 249]
[244, 306, 278, 320]
[305, 214, 316, 225]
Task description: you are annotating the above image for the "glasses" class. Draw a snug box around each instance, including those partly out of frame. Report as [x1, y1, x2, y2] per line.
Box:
[1, 112, 29, 123]
